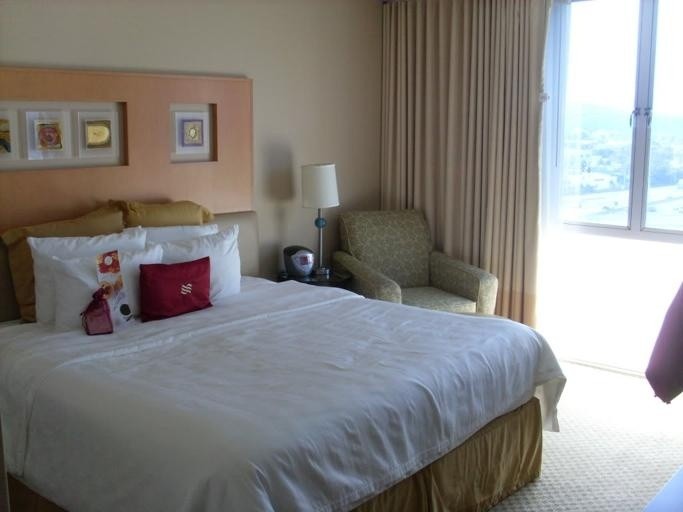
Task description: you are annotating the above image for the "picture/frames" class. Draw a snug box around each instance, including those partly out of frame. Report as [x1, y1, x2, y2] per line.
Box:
[77, 111, 117, 159]
[0, 110, 20, 161]
[25, 110, 71, 160]
[175, 111, 209, 155]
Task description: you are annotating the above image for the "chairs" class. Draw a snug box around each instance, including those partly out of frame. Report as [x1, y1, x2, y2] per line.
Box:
[332, 208, 498, 316]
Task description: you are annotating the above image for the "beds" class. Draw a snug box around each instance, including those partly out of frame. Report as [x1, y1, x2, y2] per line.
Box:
[0, 212, 566, 512]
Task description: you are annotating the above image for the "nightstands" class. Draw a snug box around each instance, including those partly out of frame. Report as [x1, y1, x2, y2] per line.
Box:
[278, 266, 352, 290]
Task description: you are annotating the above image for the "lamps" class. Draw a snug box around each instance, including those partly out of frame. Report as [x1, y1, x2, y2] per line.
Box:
[301, 163, 341, 275]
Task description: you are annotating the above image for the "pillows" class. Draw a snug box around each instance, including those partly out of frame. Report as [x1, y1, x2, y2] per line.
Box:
[2, 198, 240, 334]
